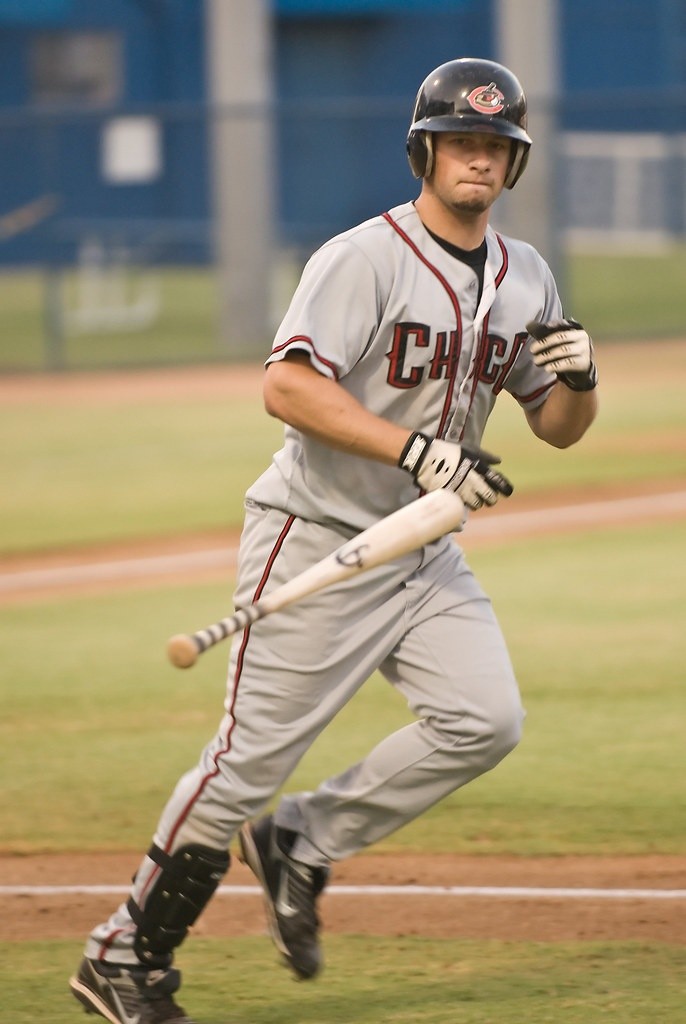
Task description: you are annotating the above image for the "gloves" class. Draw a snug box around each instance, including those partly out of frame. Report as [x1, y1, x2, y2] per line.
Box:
[398, 430, 513, 511]
[526, 319, 599, 391]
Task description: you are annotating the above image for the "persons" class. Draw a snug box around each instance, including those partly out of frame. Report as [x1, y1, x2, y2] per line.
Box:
[66, 60, 598, 1024]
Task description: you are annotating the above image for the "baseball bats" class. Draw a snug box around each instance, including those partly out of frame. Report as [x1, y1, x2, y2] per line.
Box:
[166, 487, 467, 666]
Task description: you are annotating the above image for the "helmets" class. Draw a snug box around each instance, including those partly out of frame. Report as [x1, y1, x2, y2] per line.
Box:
[406, 58, 533, 190]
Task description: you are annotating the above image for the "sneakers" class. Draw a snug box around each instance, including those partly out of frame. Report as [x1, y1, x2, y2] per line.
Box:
[69, 957, 199, 1024]
[238, 812, 328, 977]
